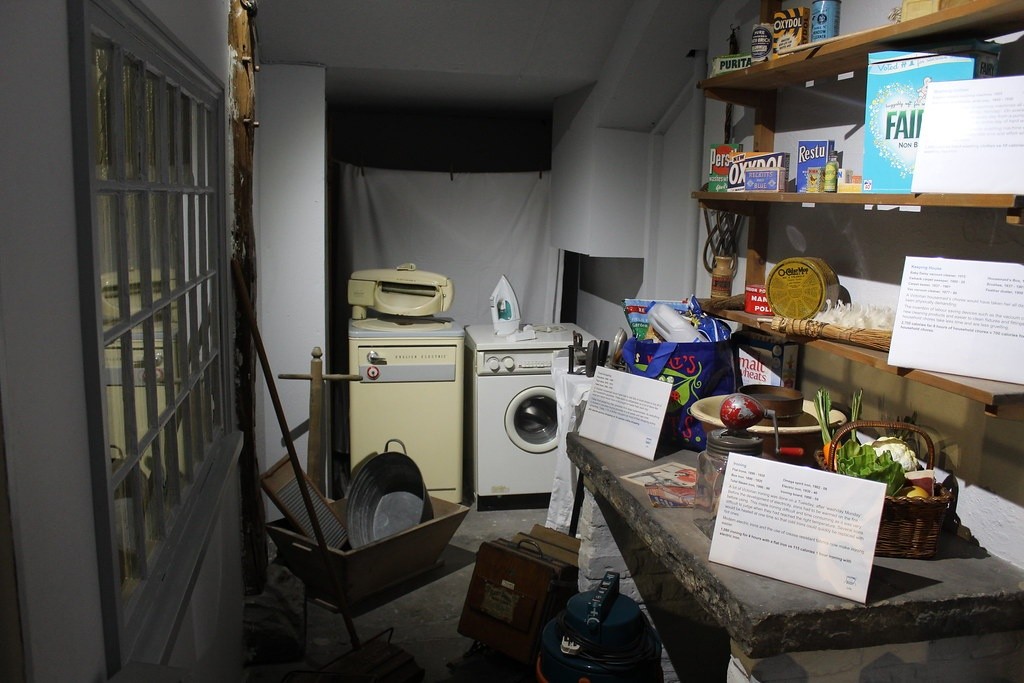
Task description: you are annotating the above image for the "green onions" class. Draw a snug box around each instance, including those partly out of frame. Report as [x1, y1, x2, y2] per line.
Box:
[887, 412, 917, 441]
[850, 388, 862, 442]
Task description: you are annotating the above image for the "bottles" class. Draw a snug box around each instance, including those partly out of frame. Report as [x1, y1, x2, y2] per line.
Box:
[691, 429, 773, 540]
[712, 256, 733, 299]
[823, 150, 839, 192]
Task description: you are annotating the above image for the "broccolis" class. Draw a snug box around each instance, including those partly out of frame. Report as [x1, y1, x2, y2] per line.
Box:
[861, 437, 919, 473]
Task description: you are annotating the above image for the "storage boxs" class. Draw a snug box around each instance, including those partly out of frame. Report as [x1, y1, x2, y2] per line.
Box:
[743, 283, 774, 318]
[764, 255, 841, 321]
[793, 137, 829, 193]
[770, 5, 810, 63]
[742, 165, 787, 194]
[707, 142, 744, 194]
[730, 328, 803, 390]
[706, 52, 754, 78]
[859, 36, 1003, 194]
[265, 496, 472, 615]
[726, 150, 790, 194]
[805, 165, 823, 193]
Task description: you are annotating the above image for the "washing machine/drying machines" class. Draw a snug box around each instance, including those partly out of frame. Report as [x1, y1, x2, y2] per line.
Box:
[464, 321, 604, 510]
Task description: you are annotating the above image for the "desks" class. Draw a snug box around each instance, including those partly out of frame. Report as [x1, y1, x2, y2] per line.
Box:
[544, 348, 649, 550]
[563, 423, 1024, 683]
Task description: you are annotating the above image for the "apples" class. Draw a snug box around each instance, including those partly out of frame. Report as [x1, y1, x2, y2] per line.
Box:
[894, 485, 929, 499]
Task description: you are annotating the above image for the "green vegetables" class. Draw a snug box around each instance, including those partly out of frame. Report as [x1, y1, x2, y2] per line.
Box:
[835, 440, 906, 498]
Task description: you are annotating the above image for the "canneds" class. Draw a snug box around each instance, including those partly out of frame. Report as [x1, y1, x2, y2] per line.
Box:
[711, 256, 733, 299]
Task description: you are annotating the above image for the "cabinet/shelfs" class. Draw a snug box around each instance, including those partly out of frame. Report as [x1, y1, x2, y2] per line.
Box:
[651, 0, 1024, 426]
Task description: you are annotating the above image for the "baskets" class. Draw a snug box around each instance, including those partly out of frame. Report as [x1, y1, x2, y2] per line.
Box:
[814, 421, 951, 559]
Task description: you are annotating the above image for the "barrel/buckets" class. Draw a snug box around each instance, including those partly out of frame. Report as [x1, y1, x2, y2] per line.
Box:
[347, 439, 434, 549]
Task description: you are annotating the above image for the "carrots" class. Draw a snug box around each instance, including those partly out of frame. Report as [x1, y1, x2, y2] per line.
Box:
[823, 440, 841, 471]
